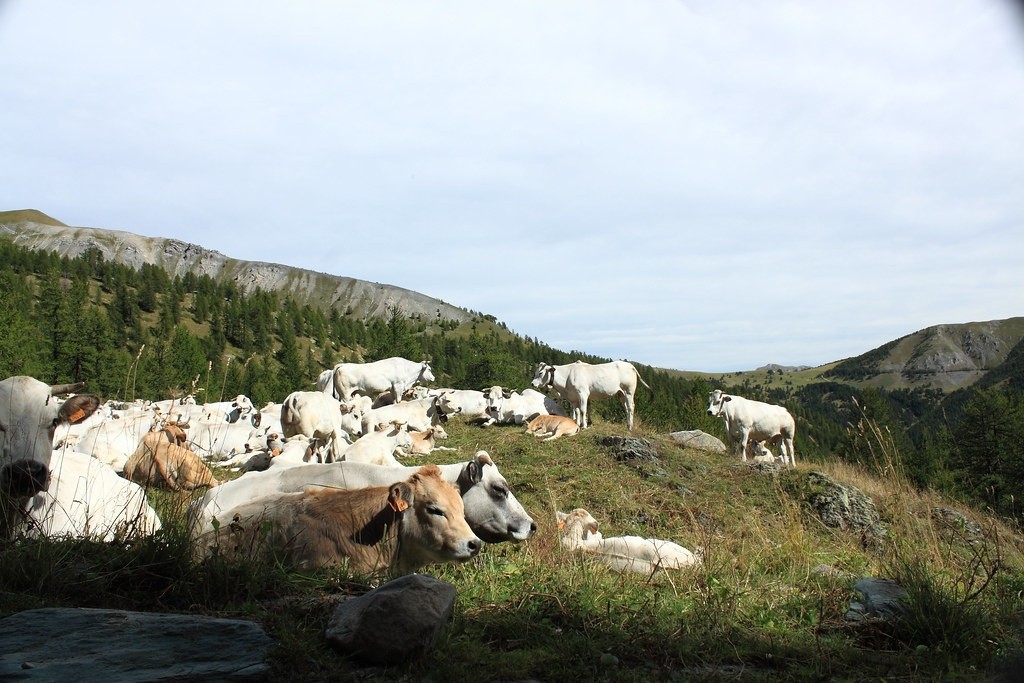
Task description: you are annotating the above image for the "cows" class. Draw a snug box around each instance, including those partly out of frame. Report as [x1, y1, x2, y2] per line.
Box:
[534, 361, 656, 432]
[705, 389, 799, 469]
[0, 357, 583, 583]
[555, 509, 705, 578]
